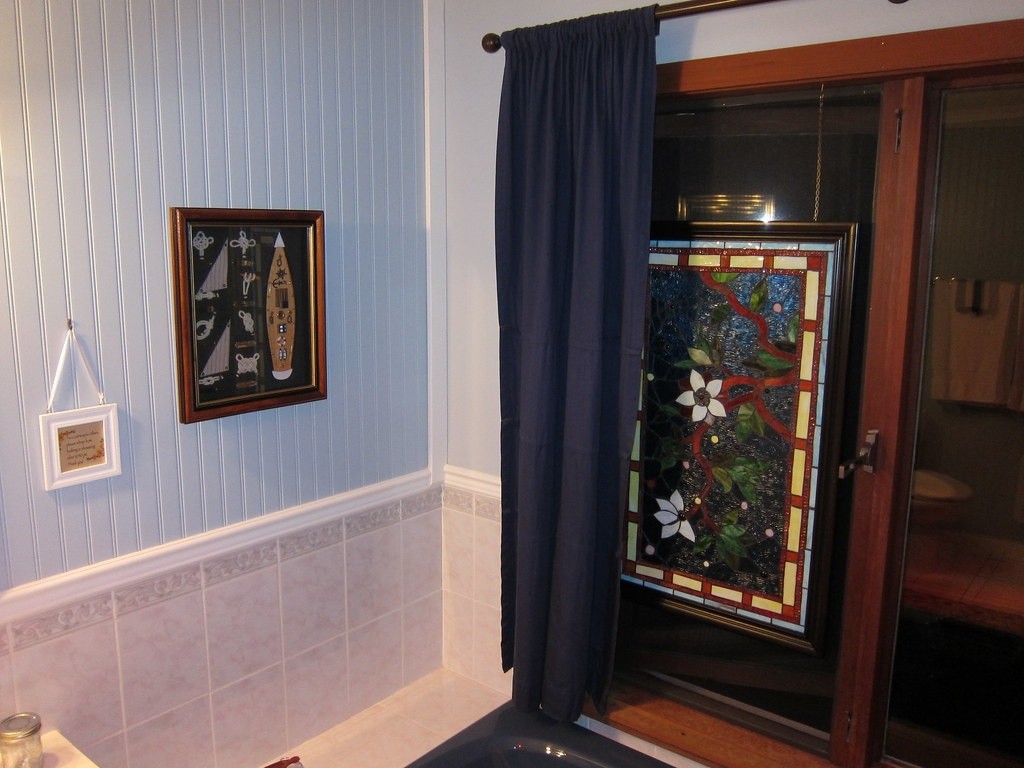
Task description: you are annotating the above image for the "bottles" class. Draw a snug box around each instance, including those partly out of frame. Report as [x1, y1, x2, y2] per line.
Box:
[0, 712, 44, 768]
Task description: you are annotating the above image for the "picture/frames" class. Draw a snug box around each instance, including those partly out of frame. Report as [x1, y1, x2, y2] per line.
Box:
[39, 403, 122, 492]
[620, 219, 859, 658]
[170, 207, 327, 424]
